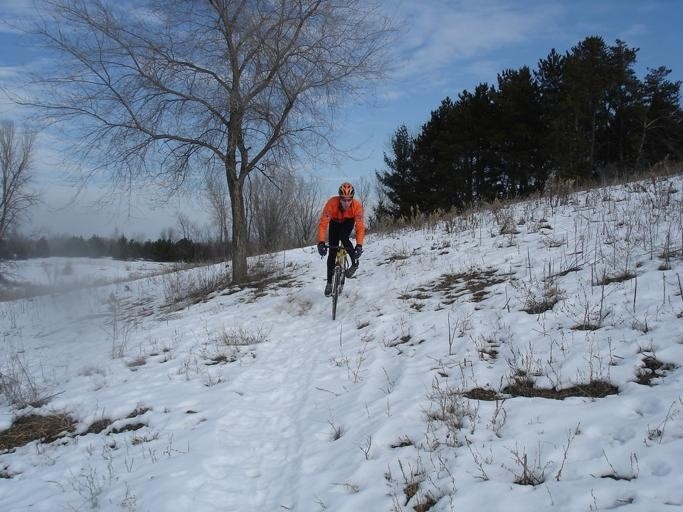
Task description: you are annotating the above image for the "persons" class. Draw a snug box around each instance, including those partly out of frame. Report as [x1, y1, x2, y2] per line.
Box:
[315, 180, 365, 298]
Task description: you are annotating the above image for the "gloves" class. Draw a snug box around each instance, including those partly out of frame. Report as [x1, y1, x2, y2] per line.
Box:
[355, 245, 362, 259]
[319, 242, 327, 255]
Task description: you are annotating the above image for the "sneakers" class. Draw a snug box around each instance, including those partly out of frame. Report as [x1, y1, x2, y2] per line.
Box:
[346, 265, 358, 278]
[325, 286, 332, 296]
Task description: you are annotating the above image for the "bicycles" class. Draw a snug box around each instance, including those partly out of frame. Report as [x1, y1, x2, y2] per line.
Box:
[312, 243, 361, 322]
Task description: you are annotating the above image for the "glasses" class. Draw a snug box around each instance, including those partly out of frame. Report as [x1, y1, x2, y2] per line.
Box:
[342, 199, 353, 201]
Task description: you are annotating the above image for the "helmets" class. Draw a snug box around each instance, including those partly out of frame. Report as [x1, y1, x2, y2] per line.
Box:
[339, 183, 354, 199]
